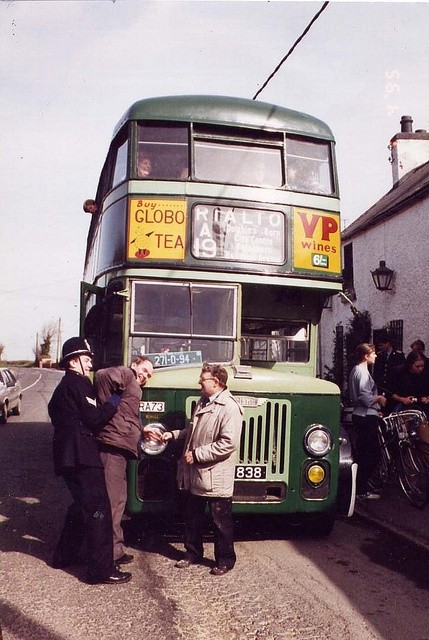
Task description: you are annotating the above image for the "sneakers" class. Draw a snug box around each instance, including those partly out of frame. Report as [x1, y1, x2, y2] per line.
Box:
[355, 492, 381, 504]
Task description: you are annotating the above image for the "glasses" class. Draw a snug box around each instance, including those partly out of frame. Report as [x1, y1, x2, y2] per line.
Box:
[199, 377, 214, 383]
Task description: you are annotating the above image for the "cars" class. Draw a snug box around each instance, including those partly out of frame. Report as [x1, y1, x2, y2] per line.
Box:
[0, 368, 23, 424]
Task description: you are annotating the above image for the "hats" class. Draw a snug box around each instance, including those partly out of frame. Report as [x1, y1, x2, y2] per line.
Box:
[57, 337, 93, 368]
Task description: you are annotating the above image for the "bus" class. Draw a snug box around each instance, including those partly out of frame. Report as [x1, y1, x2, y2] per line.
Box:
[80, 96, 344, 537]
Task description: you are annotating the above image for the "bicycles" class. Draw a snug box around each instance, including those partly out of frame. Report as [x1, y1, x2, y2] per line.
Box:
[388, 410, 429, 502]
[378, 412, 428, 508]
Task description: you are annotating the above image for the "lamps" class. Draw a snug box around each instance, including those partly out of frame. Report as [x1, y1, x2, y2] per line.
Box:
[369, 261, 393, 292]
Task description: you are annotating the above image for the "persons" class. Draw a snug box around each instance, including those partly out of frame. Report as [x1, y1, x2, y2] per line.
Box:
[410, 340, 428, 368]
[371, 335, 407, 411]
[387, 350, 428, 426]
[83, 199, 97, 215]
[136, 156, 152, 177]
[73, 354, 163, 569]
[341, 342, 386, 502]
[159, 363, 245, 576]
[48, 336, 133, 586]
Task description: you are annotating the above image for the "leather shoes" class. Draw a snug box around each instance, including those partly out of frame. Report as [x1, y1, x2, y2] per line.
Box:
[52, 557, 73, 567]
[113, 553, 133, 563]
[101, 569, 131, 584]
[175, 552, 193, 568]
[211, 564, 229, 575]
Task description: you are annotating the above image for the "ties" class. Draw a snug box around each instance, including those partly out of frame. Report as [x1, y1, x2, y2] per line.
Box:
[201, 397, 209, 409]
